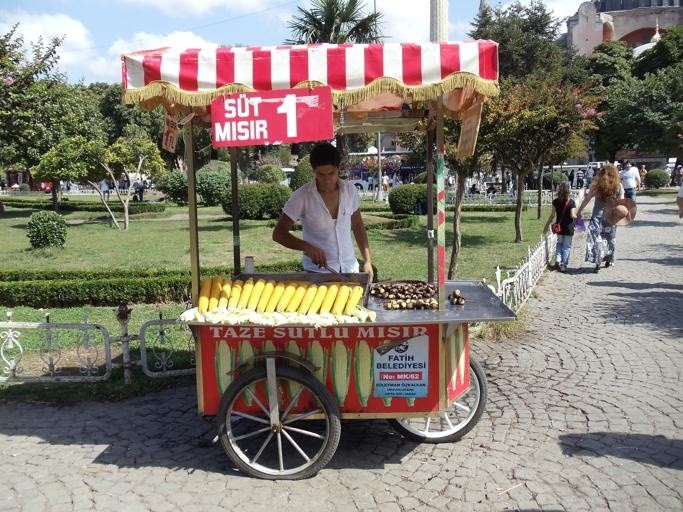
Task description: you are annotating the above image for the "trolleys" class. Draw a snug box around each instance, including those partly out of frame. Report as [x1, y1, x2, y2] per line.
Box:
[119, 39, 519, 482]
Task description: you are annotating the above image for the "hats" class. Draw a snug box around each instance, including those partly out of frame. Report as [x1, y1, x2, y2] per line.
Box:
[604, 198, 637, 226]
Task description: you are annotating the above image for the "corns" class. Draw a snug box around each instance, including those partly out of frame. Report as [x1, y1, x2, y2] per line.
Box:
[449, 323, 466, 391]
[331, 339, 352, 409]
[381, 338, 393, 407]
[198, 276, 364, 316]
[238, 339, 258, 407]
[261, 338, 281, 405]
[354, 339, 374, 409]
[284, 339, 305, 408]
[405, 397, 417, 408]
[214, 338, 235, 398]
[307, 338, 328, 409]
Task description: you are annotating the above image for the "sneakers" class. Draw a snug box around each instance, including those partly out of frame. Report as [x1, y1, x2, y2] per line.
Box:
[555, 261, 564, 272]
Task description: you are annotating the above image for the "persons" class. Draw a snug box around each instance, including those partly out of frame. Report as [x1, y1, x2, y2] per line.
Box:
[396, 177, 400, 185]
[344, 171, 349, 178]
[677, 183, 683, 218]
[273, 143, 374, 283]
[665, 164, 683, 188]
[526, 160, 647, 189]
[382, 171, 389, 191]
[133, 178, 143, 193]
[505, 172, 511, 190]
[448, 175, 455, 187]
[620, 160, 640, 201]
[576, 163, 624, 273]
[492, 173, 499, 191]
[469, 184, 477, 194]
[543, 182, 584, 272]
[100, 178, 109, 201]
[368, 176, 373, 190]
[487, 184, 497, 194]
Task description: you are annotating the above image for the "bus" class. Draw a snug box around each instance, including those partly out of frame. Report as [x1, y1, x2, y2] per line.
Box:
[343, 150, 427, 191]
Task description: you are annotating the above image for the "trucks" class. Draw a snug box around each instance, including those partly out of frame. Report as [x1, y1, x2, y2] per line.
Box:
[585, 157, 677, 178]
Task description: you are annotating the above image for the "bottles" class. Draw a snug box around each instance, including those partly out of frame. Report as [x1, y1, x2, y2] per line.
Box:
[244, 256, 254, 274]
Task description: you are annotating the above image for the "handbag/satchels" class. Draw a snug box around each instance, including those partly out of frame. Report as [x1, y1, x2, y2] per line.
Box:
[552, 224, 560, 234]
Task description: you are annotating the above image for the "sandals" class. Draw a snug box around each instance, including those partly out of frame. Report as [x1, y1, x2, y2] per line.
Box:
[605, 256, 613, 268]
[594, 264, 601, 273]
